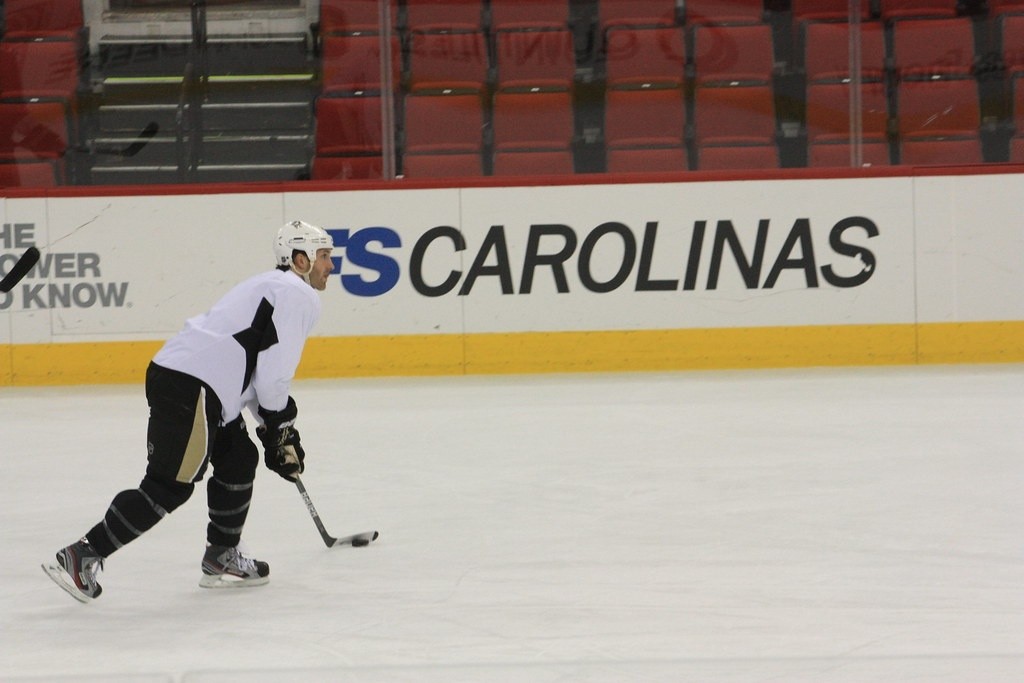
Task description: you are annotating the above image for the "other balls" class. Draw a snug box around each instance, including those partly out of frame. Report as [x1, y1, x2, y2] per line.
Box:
[352, 538, 369, 546]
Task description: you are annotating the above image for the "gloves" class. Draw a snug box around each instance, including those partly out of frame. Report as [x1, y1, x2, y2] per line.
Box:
[256, 396, 305, 483]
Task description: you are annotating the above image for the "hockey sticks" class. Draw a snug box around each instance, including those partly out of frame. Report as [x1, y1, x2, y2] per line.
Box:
[292, 469, 380, 548]
[0, 245, 41, 294]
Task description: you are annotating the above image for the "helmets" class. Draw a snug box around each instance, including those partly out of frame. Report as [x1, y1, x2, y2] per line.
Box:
[273, 221, 333, 267]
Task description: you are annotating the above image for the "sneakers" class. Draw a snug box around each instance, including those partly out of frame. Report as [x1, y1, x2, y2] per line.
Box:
[41, 536, 102, 604]
[199, 544, 270, 588]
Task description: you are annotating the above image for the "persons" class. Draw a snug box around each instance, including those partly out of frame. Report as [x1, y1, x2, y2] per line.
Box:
[42, 220, 335, 604]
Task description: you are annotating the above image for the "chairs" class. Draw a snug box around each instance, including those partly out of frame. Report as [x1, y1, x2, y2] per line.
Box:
[317, 1, 1024, 173]
[1, 1, 89, 186]
